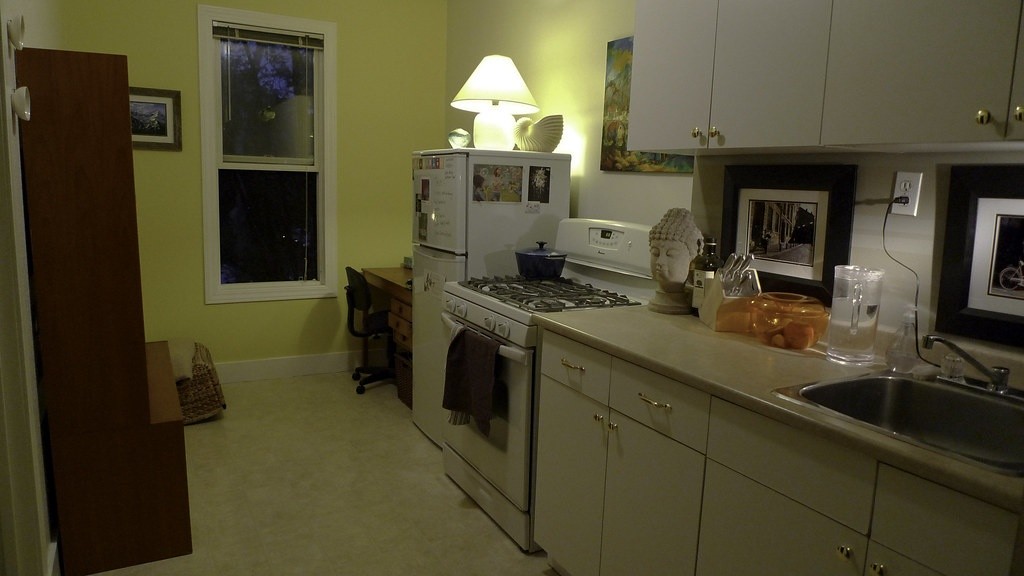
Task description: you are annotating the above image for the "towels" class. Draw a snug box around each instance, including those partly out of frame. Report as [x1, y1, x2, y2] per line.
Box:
[441, 324, 503, 438]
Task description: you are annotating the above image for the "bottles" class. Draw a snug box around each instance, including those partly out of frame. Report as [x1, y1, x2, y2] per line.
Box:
[692, 237, 724, 318]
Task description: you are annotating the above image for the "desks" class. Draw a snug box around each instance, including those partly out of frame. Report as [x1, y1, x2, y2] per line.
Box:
[361, 268, 412, 352]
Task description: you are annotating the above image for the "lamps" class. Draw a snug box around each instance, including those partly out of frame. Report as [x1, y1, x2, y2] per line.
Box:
[450, 55, 540, 151]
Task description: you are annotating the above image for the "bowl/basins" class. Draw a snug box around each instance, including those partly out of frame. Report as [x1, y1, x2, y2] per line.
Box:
[747, 292, 831, 350]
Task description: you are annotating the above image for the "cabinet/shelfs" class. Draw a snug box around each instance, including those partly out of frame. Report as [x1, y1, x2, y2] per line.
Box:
[530, 0, 1024, 576]
[13, 47, 193, 576]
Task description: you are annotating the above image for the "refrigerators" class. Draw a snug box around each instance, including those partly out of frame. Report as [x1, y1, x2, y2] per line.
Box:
[412, 150, 571, 445]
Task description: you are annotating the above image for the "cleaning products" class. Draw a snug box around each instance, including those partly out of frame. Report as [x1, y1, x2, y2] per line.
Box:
[885, 304, 918, 374]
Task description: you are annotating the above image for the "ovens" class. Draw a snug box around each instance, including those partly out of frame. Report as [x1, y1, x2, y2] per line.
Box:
[442, 309, 543, 554]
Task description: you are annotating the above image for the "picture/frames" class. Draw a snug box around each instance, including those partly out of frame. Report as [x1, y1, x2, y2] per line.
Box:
[721, 164, 858, 309]
[935, 162, 1024, 348]
[128, 87, 182, 150]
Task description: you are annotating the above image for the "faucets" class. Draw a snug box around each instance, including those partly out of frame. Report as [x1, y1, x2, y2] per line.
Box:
[921, 334, 1010, 395]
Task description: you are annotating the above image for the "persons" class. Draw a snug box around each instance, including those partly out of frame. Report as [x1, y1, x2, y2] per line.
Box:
[762, 235, 768, 254]
[649, 208, 705, 292]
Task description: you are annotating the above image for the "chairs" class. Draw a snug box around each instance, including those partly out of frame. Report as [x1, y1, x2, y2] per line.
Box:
[344, 267, 393, 394]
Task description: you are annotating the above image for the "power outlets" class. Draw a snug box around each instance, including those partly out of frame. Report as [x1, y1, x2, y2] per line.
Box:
[890, 171, 923, 217]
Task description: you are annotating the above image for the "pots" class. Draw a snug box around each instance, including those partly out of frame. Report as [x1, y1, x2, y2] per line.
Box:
[515, 242, 568, 282]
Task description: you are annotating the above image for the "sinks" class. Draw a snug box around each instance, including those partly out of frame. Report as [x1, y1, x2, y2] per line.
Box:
[769, 369, 1024, 478]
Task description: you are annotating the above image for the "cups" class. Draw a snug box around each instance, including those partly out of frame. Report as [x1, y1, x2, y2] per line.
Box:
[826, 265, 885, 367]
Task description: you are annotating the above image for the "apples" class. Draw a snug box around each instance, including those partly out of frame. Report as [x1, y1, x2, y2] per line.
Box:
[784, 321, 814, 349]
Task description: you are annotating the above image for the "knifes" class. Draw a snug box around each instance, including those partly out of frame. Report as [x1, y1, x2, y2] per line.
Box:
[725, 251, 755, 296]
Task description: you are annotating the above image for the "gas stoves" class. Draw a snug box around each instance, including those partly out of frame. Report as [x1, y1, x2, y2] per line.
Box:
[441, 218, 660, 348]
